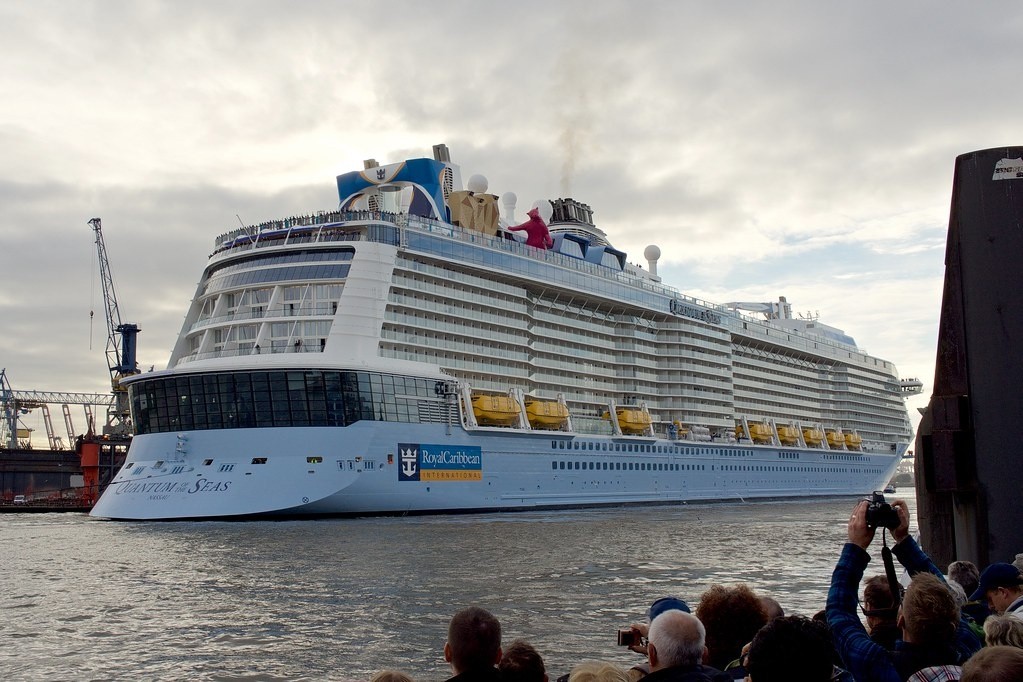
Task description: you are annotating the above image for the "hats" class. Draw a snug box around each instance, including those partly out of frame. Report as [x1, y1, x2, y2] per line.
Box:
[649, 595, 691, 622]
[1011, 553, 1023, 575]
[968, 562, 1019, 602]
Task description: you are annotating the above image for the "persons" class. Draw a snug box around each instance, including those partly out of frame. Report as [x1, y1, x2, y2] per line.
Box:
[567, 658, 632, 682]
[984, 610, 1023, 648]
[628, 609, 734, 682]
[946, 561, 990, 629]
[370, 668, 416, 682]
[644, 595, 691, 625]
[497, 641, 549, 682]
[822, 500, 983, 682]
[743, 613, 838, 682]
[978, 560, 1023, 624]
[758, 595, 785, 621]
[861, 575, 904, 647]
[438, 605, 509, 682]
[960, 644, 1023, 682]
[695, 581, 768, 668]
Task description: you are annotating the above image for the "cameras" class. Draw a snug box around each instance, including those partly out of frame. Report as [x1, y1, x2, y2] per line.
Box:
[618, 629, 642, 647]
[856, 489, 899, 529]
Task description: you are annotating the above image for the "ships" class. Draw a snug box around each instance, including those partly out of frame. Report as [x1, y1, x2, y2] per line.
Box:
[88, 144, 926, 521]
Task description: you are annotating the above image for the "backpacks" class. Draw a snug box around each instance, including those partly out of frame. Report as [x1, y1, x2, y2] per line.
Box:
[889, 641, 963, 682]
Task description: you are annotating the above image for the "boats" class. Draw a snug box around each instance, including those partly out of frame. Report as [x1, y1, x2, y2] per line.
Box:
[469, 394, 523, 427]
[801, 428, 824, 445]
[825, 431, 846, 447]
[775, 426, 801, 444]
[603, 409, 652, 435]
[843, 433, 863, 449]
[524, 399, 570, 430]
[886, 485, 896, 494]
[735, 423, 774, 443]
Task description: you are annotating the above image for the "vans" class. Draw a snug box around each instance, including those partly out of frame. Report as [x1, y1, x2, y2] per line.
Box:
[14, 495, 25, 504]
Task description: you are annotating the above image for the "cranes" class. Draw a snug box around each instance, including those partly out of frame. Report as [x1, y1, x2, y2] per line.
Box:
[87, 217, 142, 435]
[0, 368, 119, 449]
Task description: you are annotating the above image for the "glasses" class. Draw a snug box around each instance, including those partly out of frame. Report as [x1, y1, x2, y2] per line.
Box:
[899, 588, 909, 609]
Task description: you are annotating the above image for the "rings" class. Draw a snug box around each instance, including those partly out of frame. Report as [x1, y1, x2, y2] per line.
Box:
[852, 515, 856, 519]
